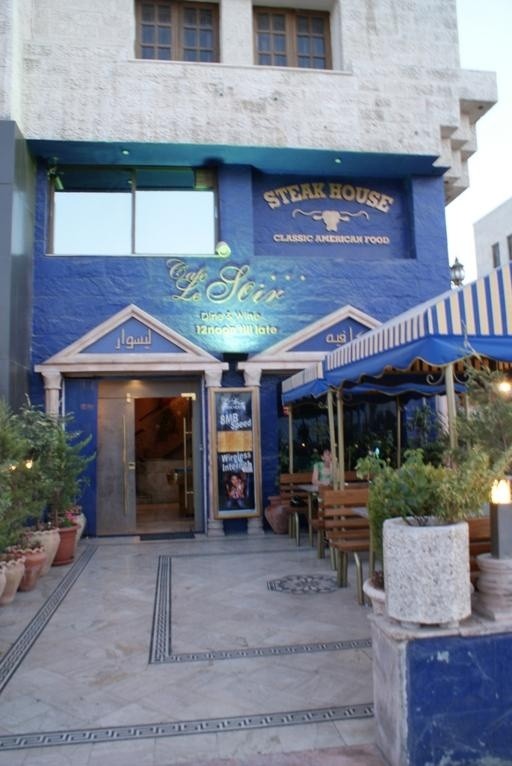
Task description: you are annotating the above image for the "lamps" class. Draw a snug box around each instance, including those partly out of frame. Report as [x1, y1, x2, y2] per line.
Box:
[487, 477, 512, 562]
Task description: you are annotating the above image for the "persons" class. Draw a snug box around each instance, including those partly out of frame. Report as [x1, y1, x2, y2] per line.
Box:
[311, 448, 341, 548]
[227, 472, 244, 499]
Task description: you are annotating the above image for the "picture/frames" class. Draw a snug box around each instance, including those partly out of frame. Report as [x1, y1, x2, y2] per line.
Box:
[208, 385, 262, 520]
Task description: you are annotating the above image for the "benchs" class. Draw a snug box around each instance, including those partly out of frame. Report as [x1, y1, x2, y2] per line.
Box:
[280, 470, 492, 615]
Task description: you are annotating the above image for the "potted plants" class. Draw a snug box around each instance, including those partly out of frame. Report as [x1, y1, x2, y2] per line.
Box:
[1, 388, 99, 608]
[354, 439, 511, 629]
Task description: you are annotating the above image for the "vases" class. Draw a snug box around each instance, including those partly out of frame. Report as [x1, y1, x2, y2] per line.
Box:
[263, 494, 291, 536]
